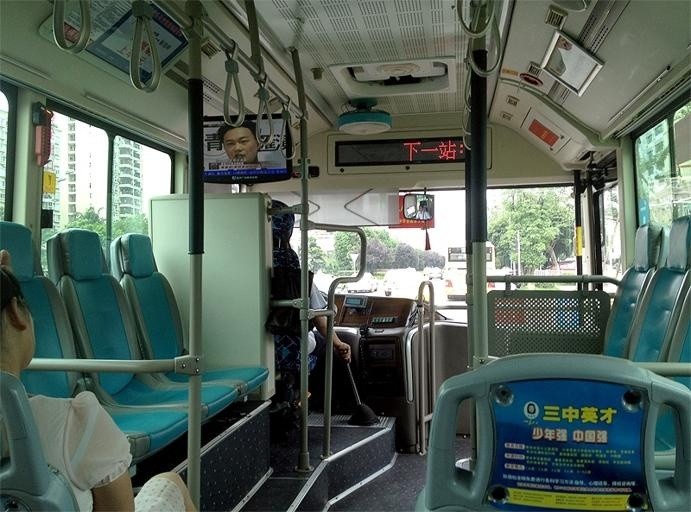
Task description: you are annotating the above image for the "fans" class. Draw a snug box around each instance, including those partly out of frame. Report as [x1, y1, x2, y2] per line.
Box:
[335, 98, 392, 135]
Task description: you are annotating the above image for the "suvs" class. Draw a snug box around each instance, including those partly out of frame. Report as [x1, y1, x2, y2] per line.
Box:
[383, 268, 428, 297]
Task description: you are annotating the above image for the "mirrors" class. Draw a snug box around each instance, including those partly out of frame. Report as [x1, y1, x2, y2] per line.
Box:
[403, 194, 434, 221]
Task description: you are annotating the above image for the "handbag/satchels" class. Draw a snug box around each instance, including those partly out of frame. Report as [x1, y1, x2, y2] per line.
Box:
[266, 237, 314, 336]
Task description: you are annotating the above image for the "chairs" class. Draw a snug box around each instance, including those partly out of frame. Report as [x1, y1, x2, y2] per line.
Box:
[110, 233, 270, 401]
[412, 351, 691, 510]
[628, 215, 690, 362]
[271, 199, 318, 372]
[603, 222, 665, 366]
[47, 228, 238, 422]
[0, 221, 189, 466]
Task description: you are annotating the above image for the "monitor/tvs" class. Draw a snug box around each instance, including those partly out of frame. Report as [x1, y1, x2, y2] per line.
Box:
[201, 112, 293, 184]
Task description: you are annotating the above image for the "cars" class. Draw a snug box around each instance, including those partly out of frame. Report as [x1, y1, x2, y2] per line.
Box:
[346, 273, 377, 293]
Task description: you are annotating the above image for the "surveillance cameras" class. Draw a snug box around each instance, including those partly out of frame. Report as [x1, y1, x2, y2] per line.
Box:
[310, 66, 325, 79]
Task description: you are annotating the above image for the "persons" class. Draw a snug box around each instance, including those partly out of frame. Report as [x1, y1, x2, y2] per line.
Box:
[217, 118, 263, 164]
[308, 281, 351, 366]
[416, 206, 430, 220]
[0, 250, 198, 512]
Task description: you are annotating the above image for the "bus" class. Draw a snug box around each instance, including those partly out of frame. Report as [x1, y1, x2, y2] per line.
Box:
[442, 239, 495, 307]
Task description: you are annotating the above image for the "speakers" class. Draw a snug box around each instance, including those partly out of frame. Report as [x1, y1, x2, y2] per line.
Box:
[551, 0, 588, 13]
